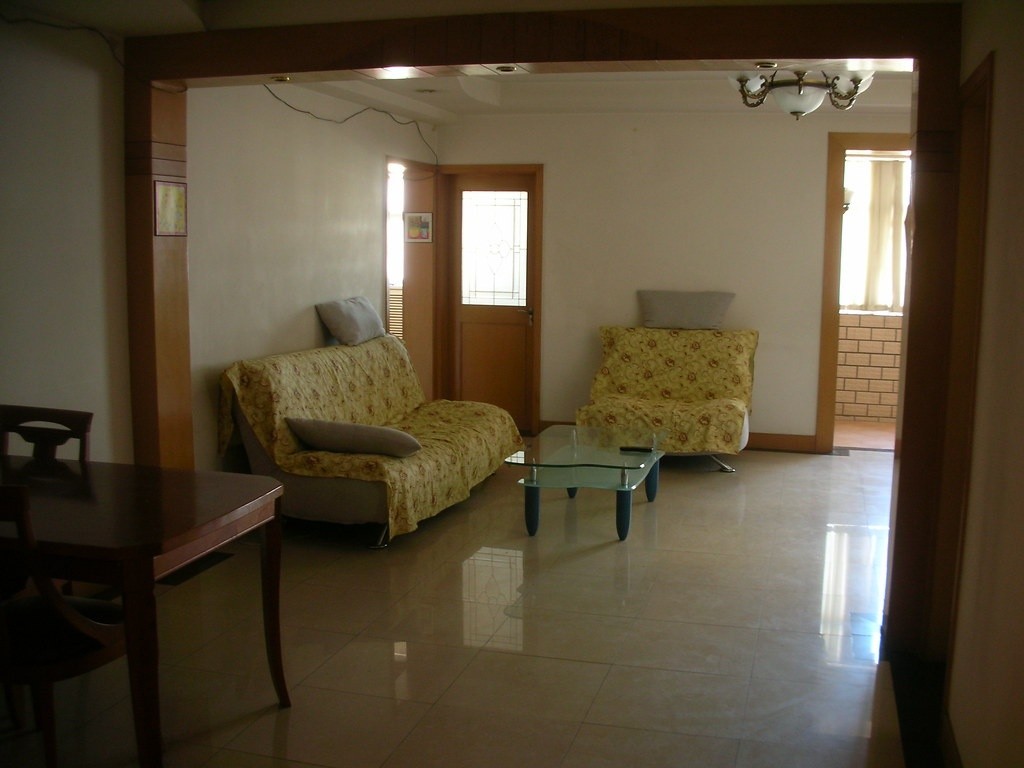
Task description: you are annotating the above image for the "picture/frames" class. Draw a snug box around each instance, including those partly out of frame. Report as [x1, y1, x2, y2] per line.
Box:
[153, 180, 189, 238]
[403, 212, 433, 243]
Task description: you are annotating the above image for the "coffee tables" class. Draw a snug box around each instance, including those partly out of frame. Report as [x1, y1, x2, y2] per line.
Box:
[504, 423, 670, 541]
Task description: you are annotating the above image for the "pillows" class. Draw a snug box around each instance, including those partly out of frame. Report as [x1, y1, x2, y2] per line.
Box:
[284, 416, 424, 458]
[315, 295, 386, 346]
[637, 289, 737, 329]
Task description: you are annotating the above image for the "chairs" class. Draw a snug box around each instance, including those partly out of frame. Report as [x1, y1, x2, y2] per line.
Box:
[0, 404, 126, 768]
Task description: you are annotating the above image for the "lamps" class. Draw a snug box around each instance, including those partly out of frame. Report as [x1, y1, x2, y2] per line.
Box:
[738, 62, 875, 121]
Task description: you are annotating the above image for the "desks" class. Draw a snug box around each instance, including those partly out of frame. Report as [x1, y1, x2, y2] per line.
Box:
[0, 455, 292, 768]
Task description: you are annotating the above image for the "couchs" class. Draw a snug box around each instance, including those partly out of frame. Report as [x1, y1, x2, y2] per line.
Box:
[574, 325, 760, 474]
[215, 332, 527, 550]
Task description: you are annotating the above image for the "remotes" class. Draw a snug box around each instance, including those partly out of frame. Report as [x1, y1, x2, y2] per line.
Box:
[620, 446, 654, 452]
